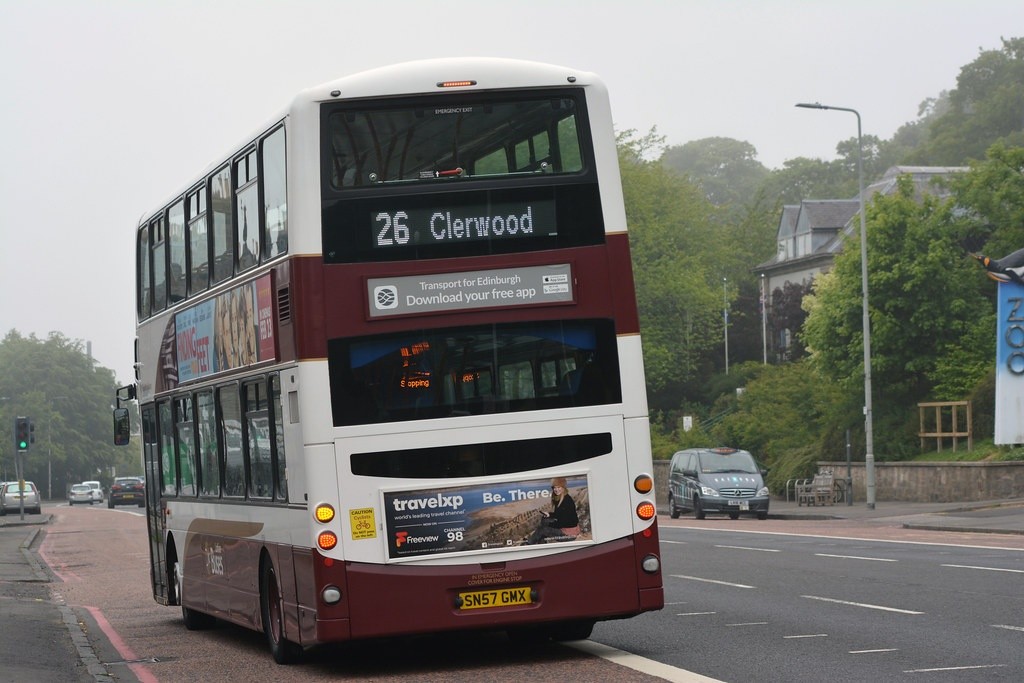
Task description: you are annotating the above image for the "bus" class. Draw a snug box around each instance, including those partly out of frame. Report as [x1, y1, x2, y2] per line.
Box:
[112, 54, 668, 666]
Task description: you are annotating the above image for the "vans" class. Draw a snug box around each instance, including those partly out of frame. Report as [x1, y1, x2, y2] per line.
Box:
[667, 446, 771, 524]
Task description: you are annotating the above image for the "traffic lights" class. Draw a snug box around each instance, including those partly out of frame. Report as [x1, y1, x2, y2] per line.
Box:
[29, 423, 35, 443]
[15, 416, 31, 453]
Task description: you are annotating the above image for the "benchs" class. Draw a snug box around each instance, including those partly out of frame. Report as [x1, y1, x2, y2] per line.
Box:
[797, 473, 835, 506]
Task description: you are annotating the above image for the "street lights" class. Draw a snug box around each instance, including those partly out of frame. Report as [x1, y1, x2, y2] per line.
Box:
[794, 101, 877, 514]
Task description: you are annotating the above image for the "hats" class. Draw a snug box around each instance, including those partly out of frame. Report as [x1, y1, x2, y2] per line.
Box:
[551, 477, 567, 489]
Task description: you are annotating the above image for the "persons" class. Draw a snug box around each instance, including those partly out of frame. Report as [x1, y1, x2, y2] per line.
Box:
[329, 355, 446, 427]
[520, 476, 580, 549]
[212, 282, 260, 373]
[144, 262, 183, 319]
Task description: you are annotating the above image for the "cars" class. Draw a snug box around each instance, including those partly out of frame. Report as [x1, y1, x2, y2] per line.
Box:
[67, 483, 94, 506]
[0, 481, 42, 517]
[82, 481, 106, 503]
[108, 476, 146, 509]
